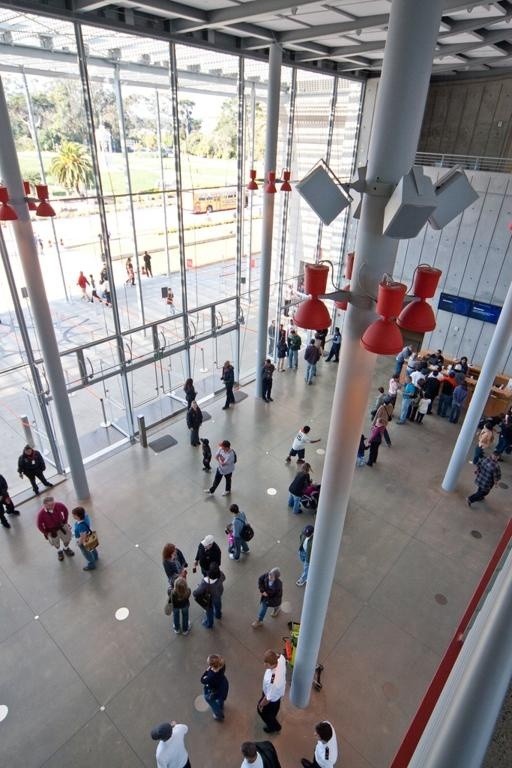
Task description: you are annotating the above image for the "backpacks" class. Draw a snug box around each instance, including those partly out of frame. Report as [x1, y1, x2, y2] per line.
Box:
[211, 673, 229, 701]
[240, 523, 255, 542]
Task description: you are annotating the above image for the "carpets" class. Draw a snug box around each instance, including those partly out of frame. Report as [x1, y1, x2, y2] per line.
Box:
[148, 432, 179, 453]
[232, 389, 249, 403]
[200, 409, 211, 422]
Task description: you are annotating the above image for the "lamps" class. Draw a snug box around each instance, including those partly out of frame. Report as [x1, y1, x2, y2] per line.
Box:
[247, 168, 265, 193]
[26, 183, 57, 220]
[360, 265, 408, 360]
[265, 170, 279, 196]
[289, 254, 352, 334]
[333, 247, 358, 312]
[279, 169, 294, 195]
[0, 178, 21, 223]
[396, 260, 446, 338]
[22, 179, 39, 211]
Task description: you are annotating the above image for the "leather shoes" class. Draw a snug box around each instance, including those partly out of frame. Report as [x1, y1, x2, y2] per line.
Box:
[302, 758, 316, 768]
[263, 725, 282, 733]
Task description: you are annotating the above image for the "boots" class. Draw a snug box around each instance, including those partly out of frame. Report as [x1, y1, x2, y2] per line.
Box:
[57, 547, 75, 560]
[270, 608, 281, 617]
[252, 620, 263, 628]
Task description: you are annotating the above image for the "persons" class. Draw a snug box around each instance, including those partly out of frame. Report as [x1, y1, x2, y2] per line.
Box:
[300, 718, 340, 768]
[467, 381, 512, 505]
[284, 426, 321, 514]
[199, 437, 214, 473]
[354, 344, 468, 466]
[150, 720, 193, 767]
[199, 653, 230, 722]
[17, 445, 54, 494]
[36, 497, 74, 564]
[0, 473, 21, 532]
[260, 319, 341, 401]
[237, 739, 283, 768]
[201, 439, 237, 499]
[185, 401, 203, 449]
[166, 287, 178, 316]
[162, 502, 315, 635]
[255, 649, 288, 735]
[75, 251, 154, 307]
[71, 505, 100, 572]
[219, 360, 236, 411]
[183, 378, 199, 411]
[33, 232, 45, 255]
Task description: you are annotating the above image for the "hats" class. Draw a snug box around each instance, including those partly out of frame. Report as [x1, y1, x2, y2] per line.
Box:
[302, 525, 314, 537]
[199, 558, 220, 574]
[201, 534, 214, 547]
[151, 723, 171, 740]
[200, 438, 231, 448]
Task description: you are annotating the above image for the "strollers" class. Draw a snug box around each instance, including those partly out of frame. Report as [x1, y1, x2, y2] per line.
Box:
[283, 619, 324, 690]
[301, 480, 320, 509]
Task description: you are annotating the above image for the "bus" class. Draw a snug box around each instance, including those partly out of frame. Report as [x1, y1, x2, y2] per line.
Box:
[181, 187, 249, 214]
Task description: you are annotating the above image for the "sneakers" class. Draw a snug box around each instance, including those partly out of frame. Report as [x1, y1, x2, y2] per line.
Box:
[191, 439, 201, 447]
[296, 459, 305, 464]
[222, 398, 235, 409]
[173, 622, 192, 635]
[1, 510, 21, 529]
[204, 488, 230, 496]
[35, 490, 40, 496]
[262, 396, 274, 403]
[44, 482, 53, 487]
[285, 457, 291, 462]
[465, 497, 471, 508]
[296, 577, 308, 586]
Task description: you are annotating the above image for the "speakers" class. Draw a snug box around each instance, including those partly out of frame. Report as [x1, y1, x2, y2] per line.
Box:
[429, 161, 480, 231]
[295, 162, 349, 227]
[349, 165, 368, 219]
[382, 173, 437, 240]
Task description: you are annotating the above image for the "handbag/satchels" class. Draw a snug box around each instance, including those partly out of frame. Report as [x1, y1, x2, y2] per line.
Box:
[80, 530, 100, 552]
[388, 414, 392, 421]
[164, 603, 172, 616]
[195, 590, 211, 611]
